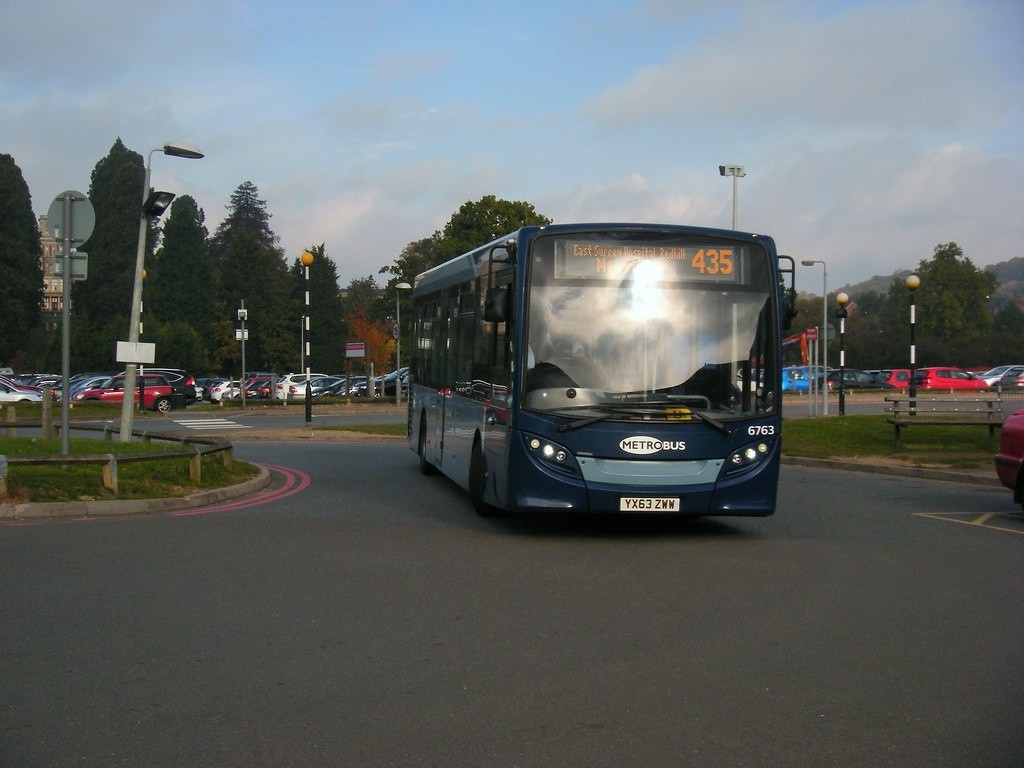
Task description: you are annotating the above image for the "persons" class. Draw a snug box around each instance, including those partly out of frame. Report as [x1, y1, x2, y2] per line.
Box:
[535, 315, 586, 367]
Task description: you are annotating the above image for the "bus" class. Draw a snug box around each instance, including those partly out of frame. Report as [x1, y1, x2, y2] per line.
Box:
[407, 222, 800, 521]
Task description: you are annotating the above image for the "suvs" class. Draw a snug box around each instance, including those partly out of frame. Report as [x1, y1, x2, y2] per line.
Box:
[72, 367, 197, 414]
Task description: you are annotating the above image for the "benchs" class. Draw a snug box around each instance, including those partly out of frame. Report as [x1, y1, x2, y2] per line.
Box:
[884, 395, 1008, 444]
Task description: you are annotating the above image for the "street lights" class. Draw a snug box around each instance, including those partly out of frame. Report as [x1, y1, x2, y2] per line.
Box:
[801, 258, 828, 417]
[395, 281, 412, 406]
[299, 253, 314, 424]
[119, 138, 206, 441]
[905, 275, 921, 415]
[720, 164, 745, 230]
[836, 292, 849, 416]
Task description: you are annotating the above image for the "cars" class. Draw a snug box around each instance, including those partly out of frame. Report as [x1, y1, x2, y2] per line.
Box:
[194, 384, 204, 402]
[0, 367, 117, 403]
[195, 367, 411, 405]
[736, 365, 1024, 394]
